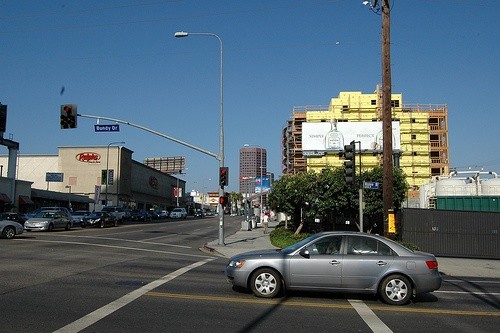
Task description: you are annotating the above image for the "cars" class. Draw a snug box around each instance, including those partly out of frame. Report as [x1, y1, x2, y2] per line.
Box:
[83, 211, 117, 228]
[225, 231, 442, 307]
[72, 210, 91, 228]
[125, 207, 170, 224]
[7, 213, 24, 223]
[0, 219, 24, 239]
[24, 210, 73, 232]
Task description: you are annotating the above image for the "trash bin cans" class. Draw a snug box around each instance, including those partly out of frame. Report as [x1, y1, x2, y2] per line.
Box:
[251, 217, 257, 229]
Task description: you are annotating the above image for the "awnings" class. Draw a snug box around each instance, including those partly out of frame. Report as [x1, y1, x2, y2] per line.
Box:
[19, 195, 34, 204]
[0, 193, 12, 203]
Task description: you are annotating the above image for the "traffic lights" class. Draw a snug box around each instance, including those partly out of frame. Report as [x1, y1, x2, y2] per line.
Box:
[344, 140, 355, 189]
[219, 167, 228, 186]
[219, 197, 227, 204]
[60, 104, 77, 129]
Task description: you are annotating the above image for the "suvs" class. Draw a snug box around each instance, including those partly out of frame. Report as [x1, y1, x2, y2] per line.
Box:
[170, 208, 188, 220]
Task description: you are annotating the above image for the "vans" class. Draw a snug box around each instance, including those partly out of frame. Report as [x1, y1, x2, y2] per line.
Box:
[101, 206, 126, 224]
[37, 207, 74, 227]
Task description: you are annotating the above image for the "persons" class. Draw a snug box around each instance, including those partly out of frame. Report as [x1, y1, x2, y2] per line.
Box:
[328, 238, 339, 254]
[262, 213, 270, 234]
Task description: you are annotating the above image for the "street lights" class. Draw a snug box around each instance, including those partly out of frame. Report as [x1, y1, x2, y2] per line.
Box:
[194, 208, 215, 218]
[105, 141, 126, 206]
[174, 31, 226, 246]
[244, 143, 270, 224]
[65, 186, 71, 207]
